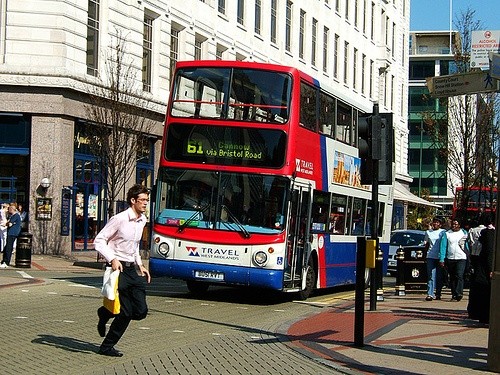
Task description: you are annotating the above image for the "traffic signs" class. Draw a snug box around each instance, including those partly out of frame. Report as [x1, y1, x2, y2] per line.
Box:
[424, 69, 498, 96]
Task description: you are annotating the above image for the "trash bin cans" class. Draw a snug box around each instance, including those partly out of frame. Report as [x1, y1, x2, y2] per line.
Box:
[405, 247, 427, 293]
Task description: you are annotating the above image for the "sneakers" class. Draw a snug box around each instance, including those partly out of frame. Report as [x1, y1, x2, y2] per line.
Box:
[0, 261, 8, 268]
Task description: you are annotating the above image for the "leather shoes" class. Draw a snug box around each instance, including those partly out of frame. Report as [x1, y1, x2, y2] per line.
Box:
[99, 347, 123, 357]
[98, 306, 106, 337]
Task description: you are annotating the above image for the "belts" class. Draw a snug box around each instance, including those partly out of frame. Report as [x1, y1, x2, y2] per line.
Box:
[106, 261, 136, 268]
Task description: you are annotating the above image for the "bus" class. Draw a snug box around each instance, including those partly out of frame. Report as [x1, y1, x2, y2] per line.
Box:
[450, 187, 496, 227]
[150, 62, 396, 300]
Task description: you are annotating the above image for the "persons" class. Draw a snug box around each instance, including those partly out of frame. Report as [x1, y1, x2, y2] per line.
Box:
[467, 212, 496, 324]
[424, 218, 442, 300]
[458, 217, 487, 277]
[175, 185, 214, 219]
[92, 184, 151, 357]
[438, 219, 471, 302]
[0, 202, 27, 269]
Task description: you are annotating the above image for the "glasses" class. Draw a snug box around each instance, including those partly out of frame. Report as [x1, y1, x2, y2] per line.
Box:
[137, 198, 149, 201]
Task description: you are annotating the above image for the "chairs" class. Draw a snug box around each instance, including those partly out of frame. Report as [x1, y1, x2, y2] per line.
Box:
[353, 220, 372, 236]
[338, 122, 351, 143]
[320, 120, 335, 141]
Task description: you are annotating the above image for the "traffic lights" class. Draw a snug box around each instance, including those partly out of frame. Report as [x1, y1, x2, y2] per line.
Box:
[357, 113, 395, 186]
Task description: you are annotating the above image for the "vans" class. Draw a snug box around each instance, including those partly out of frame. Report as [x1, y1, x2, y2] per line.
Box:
[388, 229, 427, 276]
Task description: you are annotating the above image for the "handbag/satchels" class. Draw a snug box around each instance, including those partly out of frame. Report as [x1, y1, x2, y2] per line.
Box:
[103, 267, 119, 301]
[102, 268, 120, 314]
